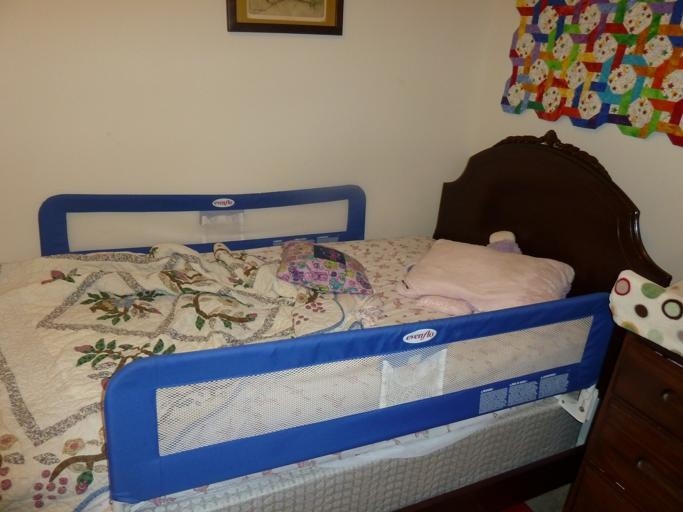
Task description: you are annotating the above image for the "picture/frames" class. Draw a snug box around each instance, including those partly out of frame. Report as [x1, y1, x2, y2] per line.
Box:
[225, 1, 343, 36]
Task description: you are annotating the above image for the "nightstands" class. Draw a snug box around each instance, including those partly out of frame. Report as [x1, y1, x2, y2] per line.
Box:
[559, 326, 683, 511]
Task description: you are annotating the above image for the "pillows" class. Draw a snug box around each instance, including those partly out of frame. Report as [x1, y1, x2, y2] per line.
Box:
[391, 239, 576, 314]
[275, 239, 373, 298]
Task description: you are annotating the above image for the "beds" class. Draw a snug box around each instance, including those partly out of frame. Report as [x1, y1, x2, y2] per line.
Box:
[0, 127, 672, 510]
